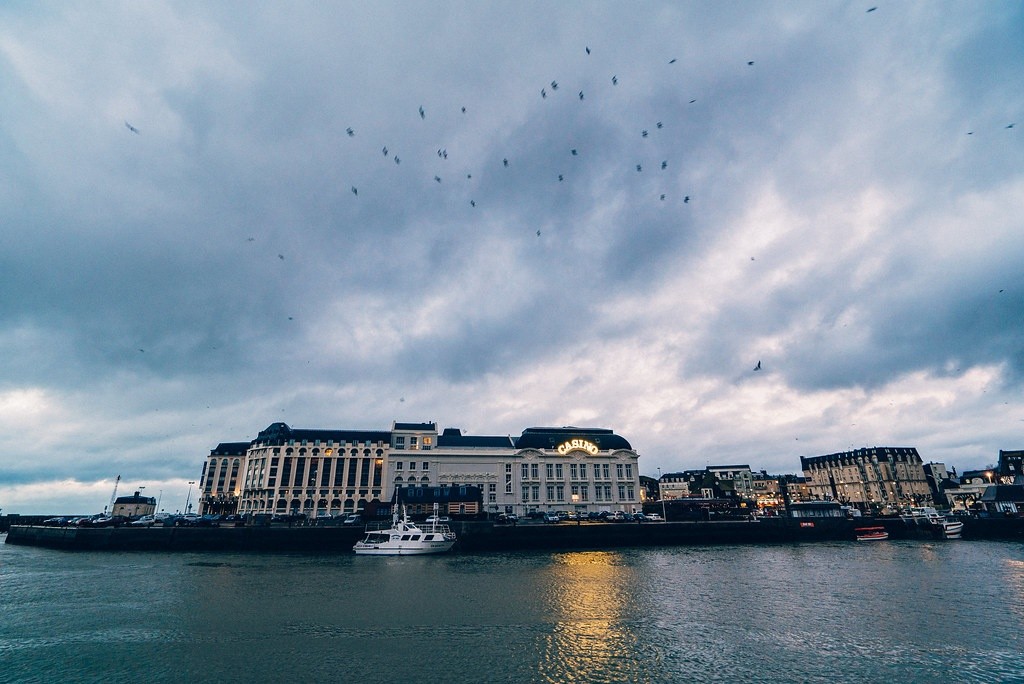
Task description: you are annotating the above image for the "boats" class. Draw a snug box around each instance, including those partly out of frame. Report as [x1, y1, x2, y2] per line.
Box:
[898, 507, 963, 542]
[352, 487, 458, 557]
[854, 527, 889, 541]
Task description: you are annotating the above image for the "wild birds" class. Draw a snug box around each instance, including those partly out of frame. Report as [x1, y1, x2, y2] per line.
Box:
[121, 3, 1020, 375]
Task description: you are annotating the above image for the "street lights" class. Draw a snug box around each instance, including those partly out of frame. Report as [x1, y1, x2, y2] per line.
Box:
[657, 467, 667, 523]
[986, 473, 991, 484]
[185, 482, 196, 514]
[156, 489, 162, 515]
[134, 486, 145, 516]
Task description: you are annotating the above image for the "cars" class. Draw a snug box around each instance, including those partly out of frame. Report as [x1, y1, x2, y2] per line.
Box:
[440, 516, 450, 522]
[498, 513, 519, 523]
[425, 515, 438, 524]
[528, 511, 663, 525]
[42, 512, 362, 527]
